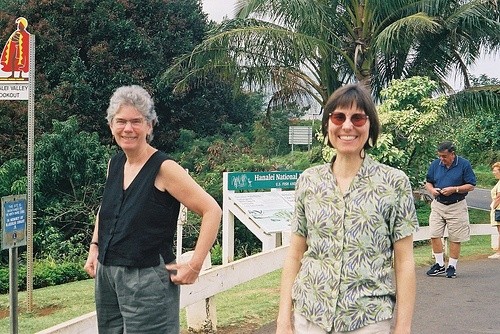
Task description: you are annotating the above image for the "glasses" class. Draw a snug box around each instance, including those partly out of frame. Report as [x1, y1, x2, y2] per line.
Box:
[328, 112, 370, 127]
[113, 117, 148, 129]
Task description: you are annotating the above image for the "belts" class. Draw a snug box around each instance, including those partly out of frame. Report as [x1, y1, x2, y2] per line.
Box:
[435, 198, 465, 206]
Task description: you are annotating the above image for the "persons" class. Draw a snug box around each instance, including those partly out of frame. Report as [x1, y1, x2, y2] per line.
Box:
[275, 85, 420, 334]
[83, 85, 223, 334]
[488, 162, 500, 259]
[424, 142, 477, 278]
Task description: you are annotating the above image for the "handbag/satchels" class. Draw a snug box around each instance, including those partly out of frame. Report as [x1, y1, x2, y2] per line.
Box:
[495, 210, 500, 221]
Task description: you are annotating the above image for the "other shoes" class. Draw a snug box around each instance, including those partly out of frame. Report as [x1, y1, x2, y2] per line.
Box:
[488, 252, 500, 260]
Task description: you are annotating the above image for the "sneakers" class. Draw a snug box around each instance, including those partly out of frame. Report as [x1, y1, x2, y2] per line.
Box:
[446, 265, 456, 279]
[426, 263, 446, 276]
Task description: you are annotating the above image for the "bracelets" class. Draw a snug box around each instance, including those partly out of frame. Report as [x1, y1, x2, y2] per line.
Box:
[90, 242, 98, 246]
[187, 261, 200, 274]
[455, 186, 459, 193]
